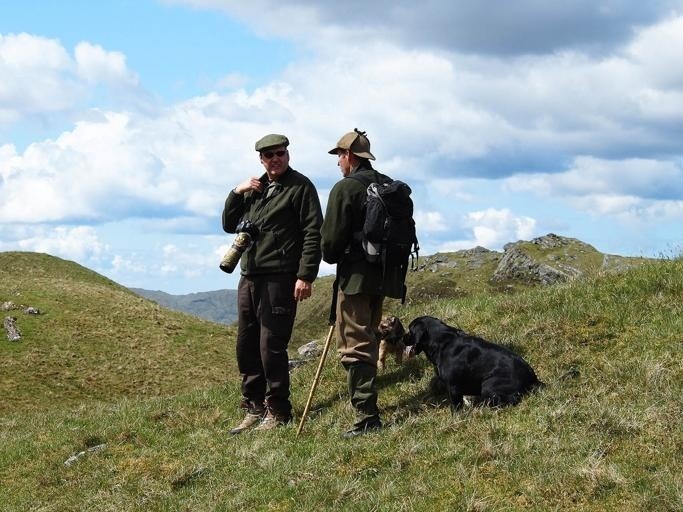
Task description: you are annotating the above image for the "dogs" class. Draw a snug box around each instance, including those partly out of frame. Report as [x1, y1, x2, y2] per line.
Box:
[401, 315, 547, 412]
[378, 314, 406, 371]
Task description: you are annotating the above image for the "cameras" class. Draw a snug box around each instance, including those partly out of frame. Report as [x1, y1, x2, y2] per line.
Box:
[219, 220, 260, 274]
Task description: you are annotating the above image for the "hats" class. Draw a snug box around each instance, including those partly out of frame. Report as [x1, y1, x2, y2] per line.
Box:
[327, 127, 376, 162]
[255, 133, 289, 151]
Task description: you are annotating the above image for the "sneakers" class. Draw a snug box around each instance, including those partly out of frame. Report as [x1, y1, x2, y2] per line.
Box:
[253, 408, 292, 433]
[343, 424, 381, 438]
[229, 400, 267, 435]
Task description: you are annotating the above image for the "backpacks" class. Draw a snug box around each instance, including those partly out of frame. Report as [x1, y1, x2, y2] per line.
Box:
[344, 174, 416, 301]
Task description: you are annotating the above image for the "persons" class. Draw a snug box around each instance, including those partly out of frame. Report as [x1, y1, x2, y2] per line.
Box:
[318, 130, 414, 441]
[221, 131, 324, 434]
